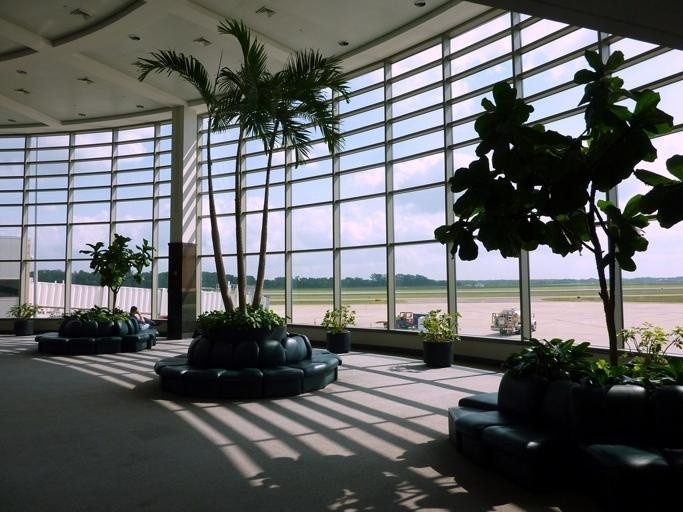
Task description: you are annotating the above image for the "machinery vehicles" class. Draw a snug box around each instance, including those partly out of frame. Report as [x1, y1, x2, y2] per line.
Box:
[382, 311, 413, 328]
[487, 306, 537, 336]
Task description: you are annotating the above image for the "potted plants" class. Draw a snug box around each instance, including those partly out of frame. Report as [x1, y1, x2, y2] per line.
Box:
[320, 307, 356, 353]
[417, 309, 462, 366]
[6, 303, 41, 336]
[615, 322, 683, 367]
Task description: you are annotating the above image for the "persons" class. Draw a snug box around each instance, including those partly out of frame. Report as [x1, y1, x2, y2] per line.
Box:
[128, 306, 160, 331]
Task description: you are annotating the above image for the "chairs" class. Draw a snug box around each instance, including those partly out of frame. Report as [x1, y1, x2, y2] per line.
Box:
[448, 368, 683, 512]
[151, 318, 342, 398]
[35, 318, 156, 359]
